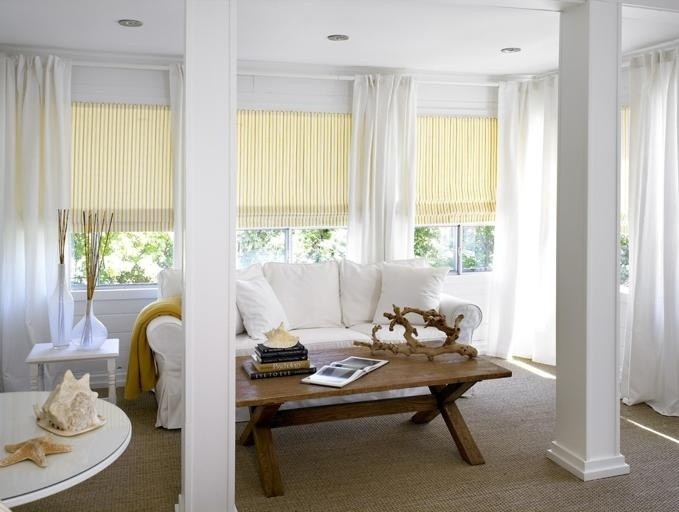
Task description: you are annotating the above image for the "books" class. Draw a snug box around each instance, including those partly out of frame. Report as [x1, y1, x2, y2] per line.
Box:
[242, 340, 317, 380]
[298, 354, 390, 388]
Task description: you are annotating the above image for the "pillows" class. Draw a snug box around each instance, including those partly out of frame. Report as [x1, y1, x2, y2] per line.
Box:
[336, 259, 381, 325]
[233, 262, 290, 336]
[256, 260, 342, 330]
[373, 260, 445, 325]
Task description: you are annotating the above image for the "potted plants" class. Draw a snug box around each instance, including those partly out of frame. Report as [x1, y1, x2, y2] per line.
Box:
[72, 212, 116, 352]
[46, 206, 77, 349]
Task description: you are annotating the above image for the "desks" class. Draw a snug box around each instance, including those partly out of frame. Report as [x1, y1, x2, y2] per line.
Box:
[235, 339, 512, 498]
[27, 339, 120, 402]
[0, 387, 133, 510]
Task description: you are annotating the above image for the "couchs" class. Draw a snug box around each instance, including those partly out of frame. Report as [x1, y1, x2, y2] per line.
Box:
[144, 260, 481, 432]
